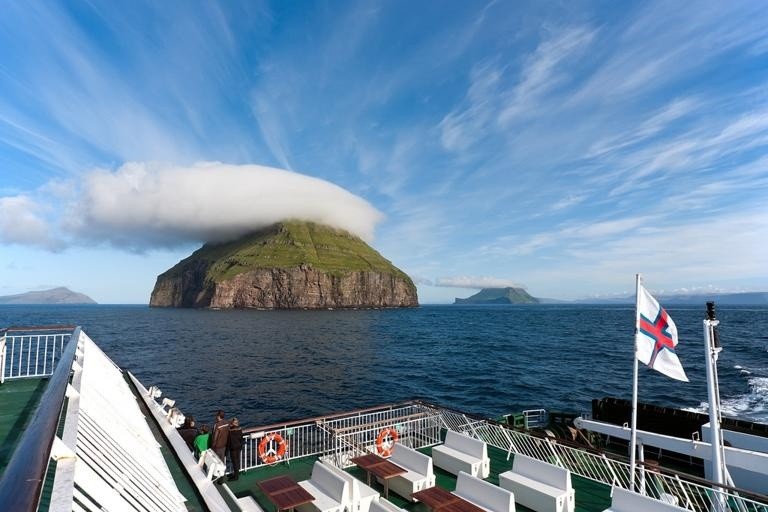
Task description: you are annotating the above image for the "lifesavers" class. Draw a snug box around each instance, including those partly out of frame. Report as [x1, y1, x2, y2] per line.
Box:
[258, 434, 286, 463]
[377, 429, 398, 456]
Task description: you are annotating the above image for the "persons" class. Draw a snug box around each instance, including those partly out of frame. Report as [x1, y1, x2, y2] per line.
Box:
[192, 424, 211, 475]
[175, 415, 199, 454]
[209, 409, 229, 485]
[226, 416, 243, 481]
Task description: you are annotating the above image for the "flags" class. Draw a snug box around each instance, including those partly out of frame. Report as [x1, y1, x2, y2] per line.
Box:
[632, 282, 690, 385]
